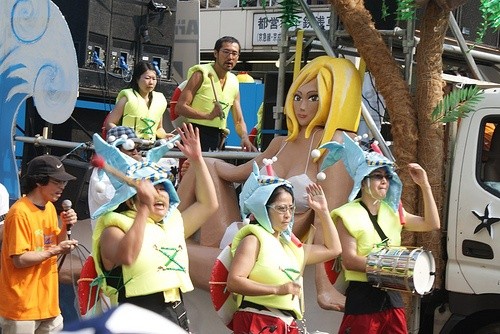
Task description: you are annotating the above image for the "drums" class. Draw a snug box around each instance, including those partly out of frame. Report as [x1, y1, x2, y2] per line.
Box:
[365, 244, 436, 298]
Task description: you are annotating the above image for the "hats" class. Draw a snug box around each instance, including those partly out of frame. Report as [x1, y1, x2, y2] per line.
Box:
[311, 131, 402, 214]
[26, 155, 77, 180]
[92, 133, 181, 218]
[106, 126, 143, 147]
[239, 158, 296, 242]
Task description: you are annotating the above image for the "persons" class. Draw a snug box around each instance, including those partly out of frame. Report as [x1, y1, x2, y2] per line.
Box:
[226, 175, 342, 334]
[88, 126, 144, 228]
[177, 56, 363, 310]
[92, 122, 219, 334]
[175, 36, 258, 152]
[104, 60, 176, 157]
[329, 157, 441, 334]
[0, 154, 79, 334]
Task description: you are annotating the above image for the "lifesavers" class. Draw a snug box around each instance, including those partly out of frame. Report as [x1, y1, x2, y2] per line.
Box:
[169, 80, 189, 130]
[77, 253, 105, 321]
[245, 124, 260, 152]
[323, 244, 351, 297]
[208, 242, 235, 332]
[101, 110, 113, 141]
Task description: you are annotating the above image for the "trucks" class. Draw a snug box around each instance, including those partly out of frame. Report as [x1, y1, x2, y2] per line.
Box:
[436, 86, 500, 334]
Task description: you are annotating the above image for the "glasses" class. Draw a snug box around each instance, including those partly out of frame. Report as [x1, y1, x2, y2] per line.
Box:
[47, 178, 68, 186]
[128, 146, 140, 152]
[364, 173, 392, 181]
[266, 204, 296, 214]
[219, 50, 240, 58]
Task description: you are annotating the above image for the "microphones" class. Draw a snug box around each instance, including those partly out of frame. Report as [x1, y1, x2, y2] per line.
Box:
[62, 199, 72, 235]
